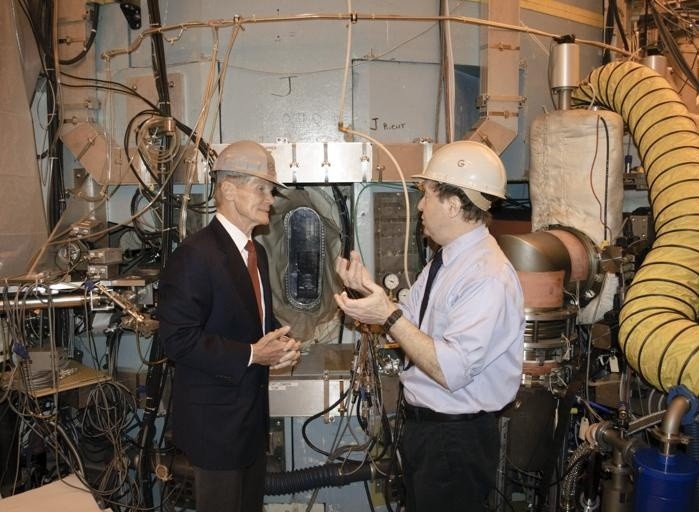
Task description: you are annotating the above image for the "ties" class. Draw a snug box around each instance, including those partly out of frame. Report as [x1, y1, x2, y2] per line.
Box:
[242, 238, 265, 331]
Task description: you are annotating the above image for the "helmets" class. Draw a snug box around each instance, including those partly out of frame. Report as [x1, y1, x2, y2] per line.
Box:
[208, 139, 292, 193]
[408, 139, 510, 201]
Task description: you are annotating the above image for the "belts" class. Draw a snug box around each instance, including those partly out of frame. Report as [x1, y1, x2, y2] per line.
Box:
[400, 407, 484, 423]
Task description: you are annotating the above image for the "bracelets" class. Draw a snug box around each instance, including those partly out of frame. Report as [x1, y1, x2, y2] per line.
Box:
[381, 309, 403, 331]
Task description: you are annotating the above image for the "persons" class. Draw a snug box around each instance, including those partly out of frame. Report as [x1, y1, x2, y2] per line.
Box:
[155, 139, 303, 512]
[333, 139, 528, 512]
